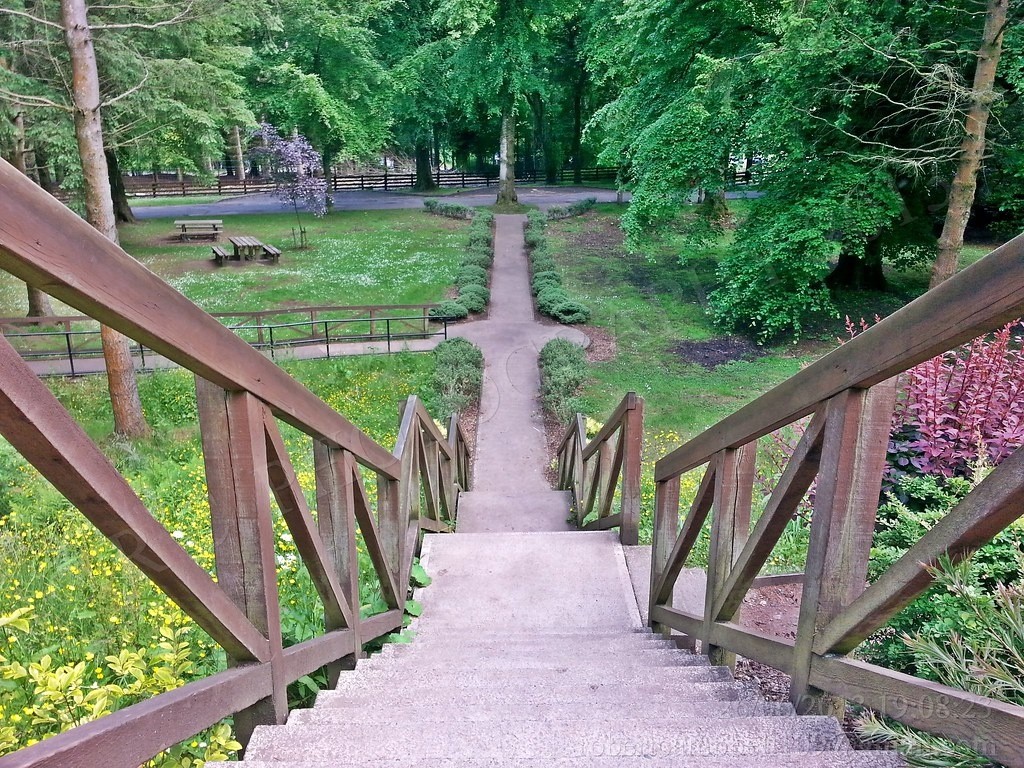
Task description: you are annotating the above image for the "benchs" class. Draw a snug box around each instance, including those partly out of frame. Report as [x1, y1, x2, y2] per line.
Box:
[211, 246, 230, 267]
[176, 225, 223, 234]
[174, 231, 223, 243]
[263, 244, 282, 263]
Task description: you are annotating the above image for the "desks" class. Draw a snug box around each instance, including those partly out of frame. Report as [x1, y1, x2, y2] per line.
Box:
[174, 220, 222, 236]
[228, 236, 265, 261]
